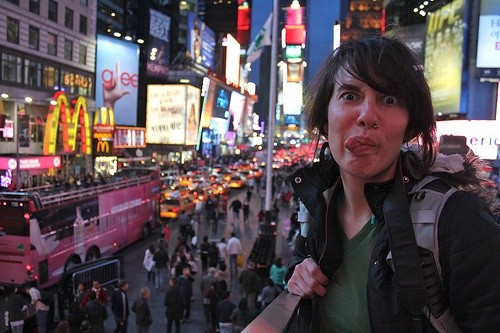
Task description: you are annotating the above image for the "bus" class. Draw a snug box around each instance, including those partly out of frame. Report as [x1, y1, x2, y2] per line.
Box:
[0, 163, 162, 293]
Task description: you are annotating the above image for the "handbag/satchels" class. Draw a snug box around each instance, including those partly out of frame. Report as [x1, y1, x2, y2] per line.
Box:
[237, 255, 244, 267]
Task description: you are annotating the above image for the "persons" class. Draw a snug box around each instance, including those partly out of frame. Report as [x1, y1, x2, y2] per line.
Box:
[0, 170, 299, 333]
[283, 35, 500, 333]
[103, 61, 131, 110]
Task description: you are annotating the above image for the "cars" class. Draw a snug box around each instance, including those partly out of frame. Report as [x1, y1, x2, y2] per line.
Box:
[162, 138, 327, 221]
